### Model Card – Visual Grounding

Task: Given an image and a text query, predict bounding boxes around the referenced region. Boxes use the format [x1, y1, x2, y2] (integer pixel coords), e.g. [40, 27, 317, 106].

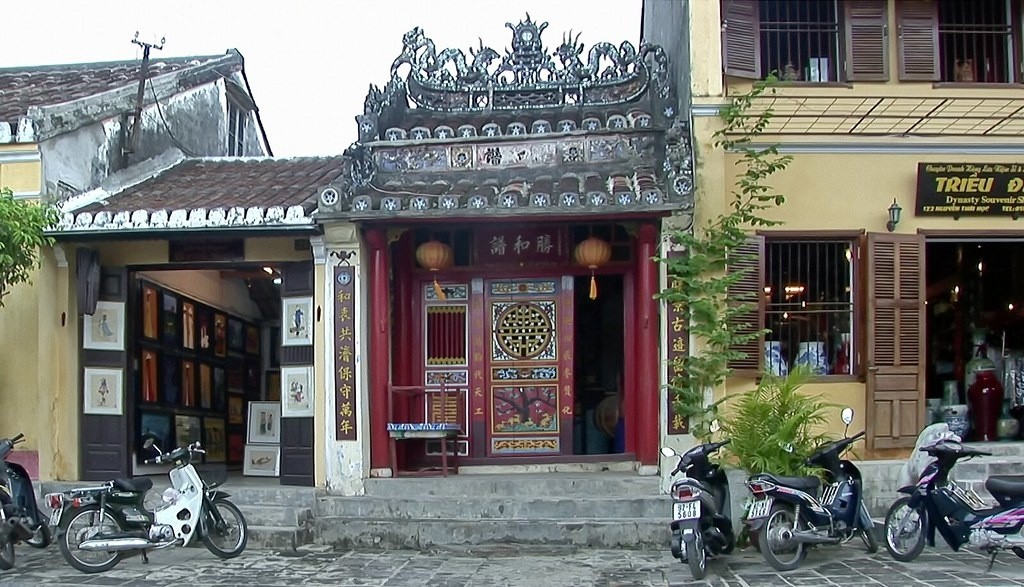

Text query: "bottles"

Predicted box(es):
[765, 333, 850, 376]
[925, 325, 1019, 443]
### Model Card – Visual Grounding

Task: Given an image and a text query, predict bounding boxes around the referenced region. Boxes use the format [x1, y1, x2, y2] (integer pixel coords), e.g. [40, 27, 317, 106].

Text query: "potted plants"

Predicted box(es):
[718, 367, 862, 555]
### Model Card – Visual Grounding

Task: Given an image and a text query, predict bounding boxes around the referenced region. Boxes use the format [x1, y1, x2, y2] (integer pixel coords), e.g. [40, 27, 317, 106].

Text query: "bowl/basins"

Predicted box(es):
[938, 405, 970, 418]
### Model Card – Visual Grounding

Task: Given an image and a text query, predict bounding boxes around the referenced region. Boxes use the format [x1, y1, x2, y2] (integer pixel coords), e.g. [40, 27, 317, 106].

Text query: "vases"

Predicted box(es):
[794, 341, 832, 374]
[836, 333, 850, 373]
[926, 329, 1024, 444]
[766, 340, 789, 377]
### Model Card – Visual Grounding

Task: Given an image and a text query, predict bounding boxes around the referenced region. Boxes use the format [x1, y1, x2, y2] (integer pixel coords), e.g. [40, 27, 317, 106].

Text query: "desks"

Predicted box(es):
[386, 380, 465, 478]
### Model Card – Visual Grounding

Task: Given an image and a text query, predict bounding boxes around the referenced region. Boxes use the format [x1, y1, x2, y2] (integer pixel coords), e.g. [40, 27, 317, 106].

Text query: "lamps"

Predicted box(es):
[885, 197, 902, 233]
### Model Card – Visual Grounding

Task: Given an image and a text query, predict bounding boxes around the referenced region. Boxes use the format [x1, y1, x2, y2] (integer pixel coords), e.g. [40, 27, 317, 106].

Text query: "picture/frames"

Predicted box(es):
[82, 278, 313, 477]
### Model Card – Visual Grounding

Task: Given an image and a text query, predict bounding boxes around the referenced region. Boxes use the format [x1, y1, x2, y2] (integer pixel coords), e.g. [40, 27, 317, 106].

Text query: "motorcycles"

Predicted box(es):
[883, 422, 1024, 573]
[0, 433, 53, 571]
[660, 417, 735, 582]
[740, 407, 879, 571]
[44, 436, 248, 574]
